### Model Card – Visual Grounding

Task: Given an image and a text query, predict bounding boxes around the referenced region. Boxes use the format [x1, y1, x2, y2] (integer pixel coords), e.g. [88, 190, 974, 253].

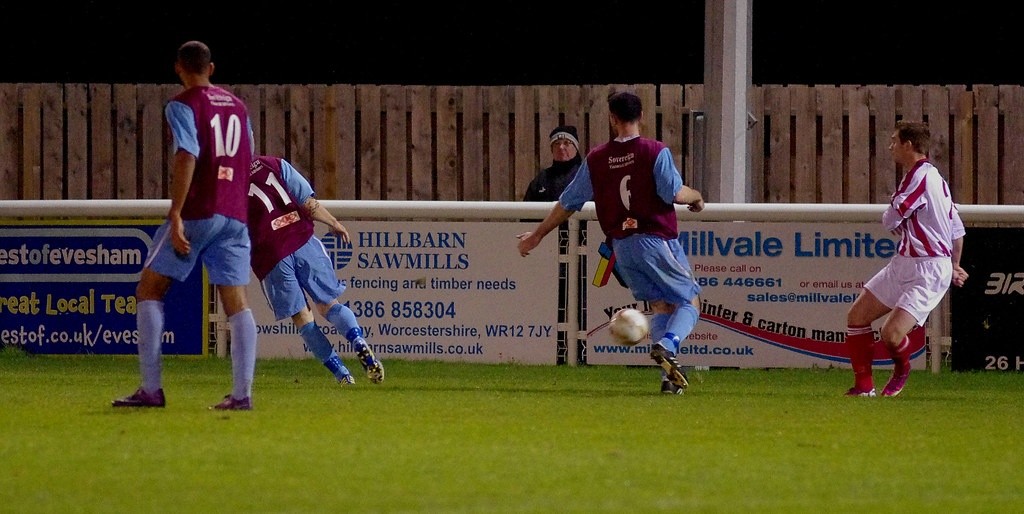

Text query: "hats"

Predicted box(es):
[549, 125, 578, 151]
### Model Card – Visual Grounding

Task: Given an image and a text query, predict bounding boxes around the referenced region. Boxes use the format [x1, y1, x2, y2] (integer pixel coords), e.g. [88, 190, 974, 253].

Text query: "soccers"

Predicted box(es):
[609, 307, 649, 348]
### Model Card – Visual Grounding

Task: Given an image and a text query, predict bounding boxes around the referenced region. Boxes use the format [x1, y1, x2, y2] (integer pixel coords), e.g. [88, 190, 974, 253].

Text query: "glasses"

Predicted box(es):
[551, 141, 573, 148]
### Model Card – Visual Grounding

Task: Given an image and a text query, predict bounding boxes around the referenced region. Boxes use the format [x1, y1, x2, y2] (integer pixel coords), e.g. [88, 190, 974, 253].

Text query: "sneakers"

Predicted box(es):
[648, 345, 689, 389]
[351, 338, 384, 383]
[843, 387, 876, 397]
[881, 364, 911, 397]
[209, 393, 252, 409]
[112, 387, 166, 409]
[338, 375, 356, 386]
[660, 379, 684, 394]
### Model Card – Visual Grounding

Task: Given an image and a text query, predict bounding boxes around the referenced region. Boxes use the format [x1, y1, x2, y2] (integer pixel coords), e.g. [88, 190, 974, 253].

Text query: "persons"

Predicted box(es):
[520, 124, 597, 365]
[248, 154, 385, 387]
[843, 119, 970, 398]
[111, 40, 258, 414]
[516, 92, 705, 395]
